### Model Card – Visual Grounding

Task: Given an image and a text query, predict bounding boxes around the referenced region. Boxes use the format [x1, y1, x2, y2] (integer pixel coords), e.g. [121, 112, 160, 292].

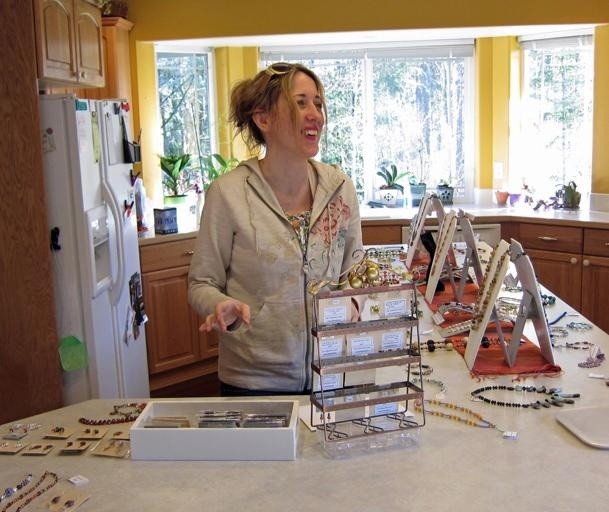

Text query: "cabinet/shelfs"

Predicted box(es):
[46, 14, 136, 140]
[32, 0, 108, 92]
[359, 217, 405, 247]
[508, 218, 609, 335]
[140, 232, 227, 393]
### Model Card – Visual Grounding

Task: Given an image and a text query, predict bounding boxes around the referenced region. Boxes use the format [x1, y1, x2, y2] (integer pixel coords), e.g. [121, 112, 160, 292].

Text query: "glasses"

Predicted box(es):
[258, 62, 290, 95]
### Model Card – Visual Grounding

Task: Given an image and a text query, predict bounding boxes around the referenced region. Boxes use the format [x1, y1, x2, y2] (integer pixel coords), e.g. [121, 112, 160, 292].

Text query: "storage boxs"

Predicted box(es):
[129, 400, 300, 462]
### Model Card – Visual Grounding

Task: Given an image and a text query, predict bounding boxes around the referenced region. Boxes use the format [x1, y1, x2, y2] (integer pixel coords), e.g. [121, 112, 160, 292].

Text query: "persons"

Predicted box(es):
[185, 61, 368, 396]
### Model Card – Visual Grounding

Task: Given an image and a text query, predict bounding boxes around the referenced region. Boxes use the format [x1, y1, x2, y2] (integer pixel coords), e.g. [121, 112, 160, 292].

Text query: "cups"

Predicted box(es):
[494, 190, 509, 210]
[508, 192, 522, 208]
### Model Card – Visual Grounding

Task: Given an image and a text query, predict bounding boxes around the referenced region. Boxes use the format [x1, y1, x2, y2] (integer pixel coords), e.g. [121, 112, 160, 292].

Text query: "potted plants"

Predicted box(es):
[157, 149, 193, 209]
[375, 162, 413, 209]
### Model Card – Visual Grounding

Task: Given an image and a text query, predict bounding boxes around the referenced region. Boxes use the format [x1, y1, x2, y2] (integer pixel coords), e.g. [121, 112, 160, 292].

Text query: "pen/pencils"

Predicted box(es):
[137, 128, 142, 144]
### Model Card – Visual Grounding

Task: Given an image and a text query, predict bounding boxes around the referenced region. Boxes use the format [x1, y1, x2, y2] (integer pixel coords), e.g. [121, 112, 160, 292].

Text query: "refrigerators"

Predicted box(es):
[38, 92, 153, 403]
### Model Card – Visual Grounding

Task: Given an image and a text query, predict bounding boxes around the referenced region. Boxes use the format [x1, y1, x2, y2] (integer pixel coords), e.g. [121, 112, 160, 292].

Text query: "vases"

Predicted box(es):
[436, 185, 456, 207]
[410, 182, 428, 208]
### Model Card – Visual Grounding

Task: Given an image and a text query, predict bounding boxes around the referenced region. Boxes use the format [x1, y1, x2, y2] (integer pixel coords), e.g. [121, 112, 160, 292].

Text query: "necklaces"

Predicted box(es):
[1, 470, 60, 511]
[364, 195, 605, 434]
[110, 401, 146, 415]
[0, 472, 33, 500]
[79, 416, 138, 427]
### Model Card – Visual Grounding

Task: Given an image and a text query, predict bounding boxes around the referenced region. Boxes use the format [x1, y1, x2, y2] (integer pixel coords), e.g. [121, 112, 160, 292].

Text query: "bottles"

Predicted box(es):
[194, 188, 205, 228]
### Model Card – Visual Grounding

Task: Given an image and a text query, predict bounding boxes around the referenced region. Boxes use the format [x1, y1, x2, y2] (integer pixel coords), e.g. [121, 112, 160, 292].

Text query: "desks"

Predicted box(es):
[0, 236, 608, 510]
